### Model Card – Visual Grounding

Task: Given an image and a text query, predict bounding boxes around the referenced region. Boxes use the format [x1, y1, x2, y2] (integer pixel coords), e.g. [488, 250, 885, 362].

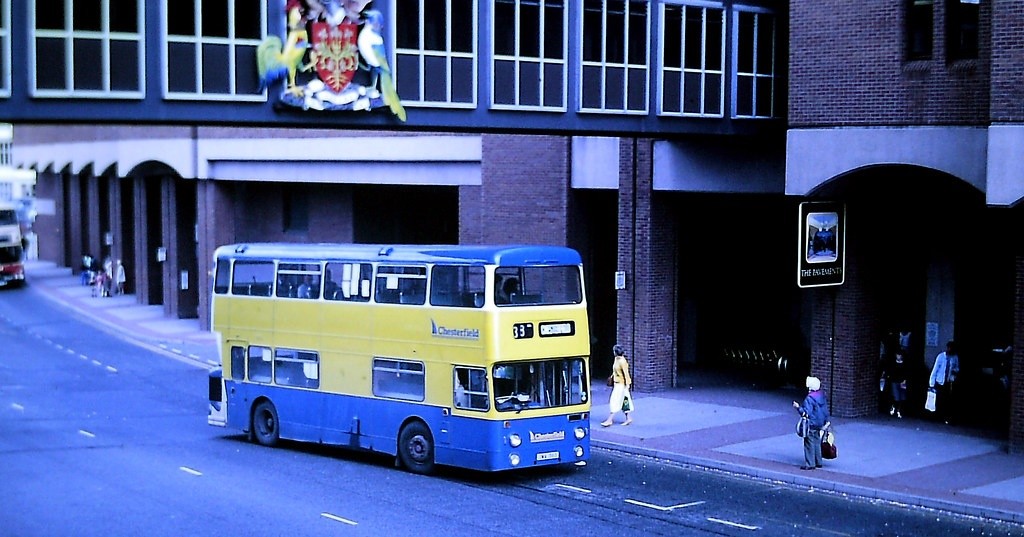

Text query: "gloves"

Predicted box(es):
[793, 401, 800, 408]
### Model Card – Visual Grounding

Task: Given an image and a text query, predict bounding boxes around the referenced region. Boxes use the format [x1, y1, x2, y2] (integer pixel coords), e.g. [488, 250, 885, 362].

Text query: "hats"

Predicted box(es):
[895, 348, 907, 357]
[805, 376, 821, 391]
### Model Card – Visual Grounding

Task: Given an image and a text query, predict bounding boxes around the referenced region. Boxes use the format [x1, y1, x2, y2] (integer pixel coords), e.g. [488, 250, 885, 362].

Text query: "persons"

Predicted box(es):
[793, 376, 831, 470]
[879, 323, 1013, 429]
[20, 235, 127, 299]
[455, 378, 473, 408]
[494, 275, 524, 307]
[298, 269, 344, 301]
[601, 345, 634, 427]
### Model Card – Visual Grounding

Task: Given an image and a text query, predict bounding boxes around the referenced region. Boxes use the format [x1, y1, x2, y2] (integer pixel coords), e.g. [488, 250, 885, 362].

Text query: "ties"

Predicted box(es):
[945, 356, 950, 382]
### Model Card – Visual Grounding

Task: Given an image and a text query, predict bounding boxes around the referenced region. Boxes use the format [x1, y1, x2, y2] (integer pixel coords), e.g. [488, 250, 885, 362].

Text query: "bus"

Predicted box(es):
[0, 208, 27, 288]
[205, 243, 593, 475]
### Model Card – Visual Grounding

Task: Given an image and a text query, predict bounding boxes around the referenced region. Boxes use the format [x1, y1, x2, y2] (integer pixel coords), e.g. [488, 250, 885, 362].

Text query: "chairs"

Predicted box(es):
[438, 291, 484, 307]
[249, 285, 255, 295]
[268, 284, 272, 296]
[333, 290, 345, 300]
[289, 282, 297, 297]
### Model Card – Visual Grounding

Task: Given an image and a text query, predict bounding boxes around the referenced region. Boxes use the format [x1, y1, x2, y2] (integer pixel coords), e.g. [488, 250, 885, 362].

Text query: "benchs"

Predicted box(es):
[400, 289, 426, 304]
[511, 289, 543, 303]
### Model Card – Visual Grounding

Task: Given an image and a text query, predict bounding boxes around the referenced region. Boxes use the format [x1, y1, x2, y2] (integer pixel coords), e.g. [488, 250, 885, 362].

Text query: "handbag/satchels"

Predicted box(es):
[607, 374, 614, 386]
[925, 388, 936, 411]
[795, 412, 810, 437]
[880, 374, 886, 391]
[622, 396, 630, 411]
[821, 430, 837, 459]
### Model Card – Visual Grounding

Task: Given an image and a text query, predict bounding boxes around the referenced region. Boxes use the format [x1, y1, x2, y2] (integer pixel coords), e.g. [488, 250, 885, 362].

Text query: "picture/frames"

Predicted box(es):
[797, 201, 846, 288]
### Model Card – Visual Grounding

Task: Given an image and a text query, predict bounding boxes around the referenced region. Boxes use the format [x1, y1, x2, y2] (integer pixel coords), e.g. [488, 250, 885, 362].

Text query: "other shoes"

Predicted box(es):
[621, 419, 632, 425]
[800, 465, 816, 470]
[815, 463, 823, 468]
[889, 404, 895, 415]
[600, 421, 614, 426]
[896, 411, 902, 420]
